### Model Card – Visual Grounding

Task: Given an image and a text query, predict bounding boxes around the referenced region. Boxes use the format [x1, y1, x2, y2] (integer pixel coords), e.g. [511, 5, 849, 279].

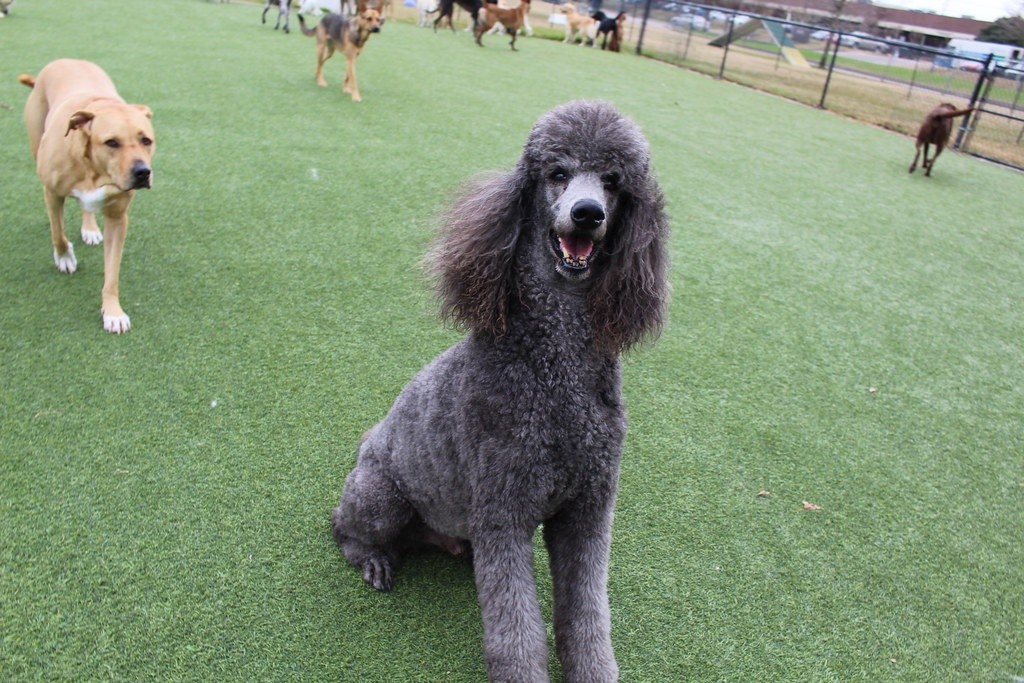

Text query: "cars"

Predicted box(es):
[670, 13, 710, 32]
[840, 32, 889, 54]
[664, 2, 844, 44]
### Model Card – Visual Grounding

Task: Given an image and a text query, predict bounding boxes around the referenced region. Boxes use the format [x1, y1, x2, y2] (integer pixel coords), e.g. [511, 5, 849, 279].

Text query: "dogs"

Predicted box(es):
[18, 59, 155, 335]
[331, 98, 671, 683]
[909, 101, 976, 178]
[262, 0, 627, 101]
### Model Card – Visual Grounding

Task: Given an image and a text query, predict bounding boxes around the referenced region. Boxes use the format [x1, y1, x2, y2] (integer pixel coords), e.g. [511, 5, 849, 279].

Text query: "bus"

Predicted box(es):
[944, 38, 1024, 78]
